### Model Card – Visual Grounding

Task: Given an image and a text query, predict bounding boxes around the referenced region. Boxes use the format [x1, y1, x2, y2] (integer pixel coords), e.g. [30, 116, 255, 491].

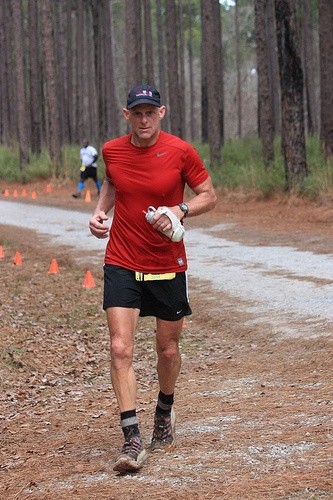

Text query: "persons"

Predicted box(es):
[72, 137, 102, 199]
[89, 84, 217, 473]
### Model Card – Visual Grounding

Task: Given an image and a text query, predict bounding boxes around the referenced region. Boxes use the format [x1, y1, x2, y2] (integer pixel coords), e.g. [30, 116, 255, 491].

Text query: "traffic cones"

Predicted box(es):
[84, 190, 91, 202]
[82, 270, 97, 288]
[4, 184, 52, 201]
[0, 245, 5, 257]
[47, 259, 62, 274]
[12, 251, 25, 266]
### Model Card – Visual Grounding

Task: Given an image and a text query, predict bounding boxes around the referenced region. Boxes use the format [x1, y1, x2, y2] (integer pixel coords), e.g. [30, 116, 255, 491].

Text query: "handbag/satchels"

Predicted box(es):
[134, 270, 177, 282]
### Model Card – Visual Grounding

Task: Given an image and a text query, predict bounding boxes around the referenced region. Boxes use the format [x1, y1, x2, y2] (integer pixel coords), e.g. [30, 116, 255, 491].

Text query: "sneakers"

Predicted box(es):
[151, 410, 177, 453]
[72, 192, 81, 199]
[113, 437, 149, 473]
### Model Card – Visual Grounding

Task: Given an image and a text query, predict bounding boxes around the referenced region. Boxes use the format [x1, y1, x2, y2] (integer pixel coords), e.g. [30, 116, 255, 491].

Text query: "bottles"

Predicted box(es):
[146, 206, 185, 242]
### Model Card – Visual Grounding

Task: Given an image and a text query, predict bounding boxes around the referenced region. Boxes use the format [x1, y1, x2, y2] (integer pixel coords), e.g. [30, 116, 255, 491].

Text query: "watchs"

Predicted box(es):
[177, 202, 189, 219]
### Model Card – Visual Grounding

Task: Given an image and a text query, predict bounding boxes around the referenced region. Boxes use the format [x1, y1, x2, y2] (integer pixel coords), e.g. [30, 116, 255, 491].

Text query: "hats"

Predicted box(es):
[126, 85, 162, 110]
